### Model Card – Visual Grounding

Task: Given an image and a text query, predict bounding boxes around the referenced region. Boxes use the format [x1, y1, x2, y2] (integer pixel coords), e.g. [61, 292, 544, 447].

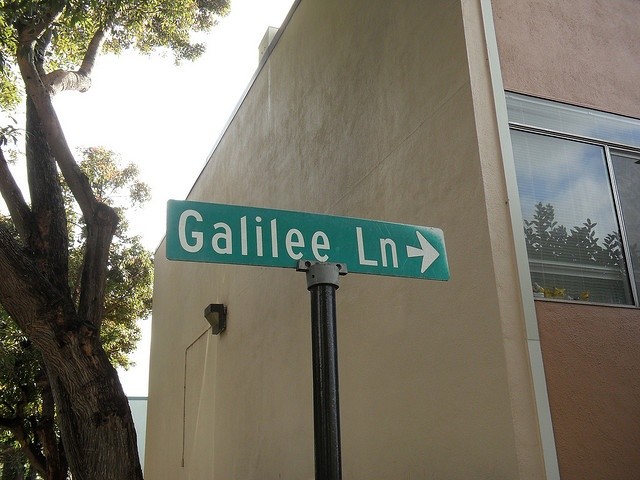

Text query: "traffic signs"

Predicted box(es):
[166, 199, 450, 282]
